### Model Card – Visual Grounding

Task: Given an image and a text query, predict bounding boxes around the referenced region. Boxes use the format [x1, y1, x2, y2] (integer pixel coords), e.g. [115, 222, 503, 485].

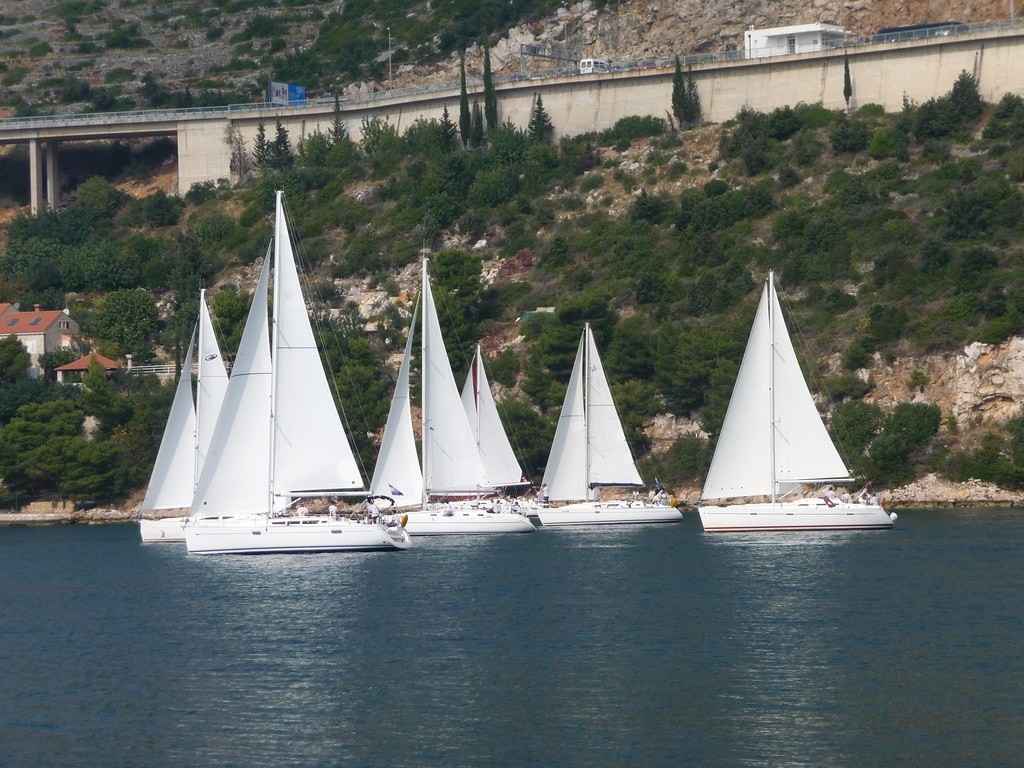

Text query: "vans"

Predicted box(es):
[578, 58, 607, 75]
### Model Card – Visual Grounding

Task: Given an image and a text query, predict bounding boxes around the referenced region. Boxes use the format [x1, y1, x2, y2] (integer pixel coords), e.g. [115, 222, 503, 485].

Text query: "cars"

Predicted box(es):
[608, 53, 718, 73]
[508, 69, 581, 83]
[842, 36, 872, 48]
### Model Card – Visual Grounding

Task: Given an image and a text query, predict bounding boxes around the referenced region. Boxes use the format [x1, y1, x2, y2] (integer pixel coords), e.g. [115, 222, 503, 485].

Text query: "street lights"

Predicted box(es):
[386, 27, 392, 90]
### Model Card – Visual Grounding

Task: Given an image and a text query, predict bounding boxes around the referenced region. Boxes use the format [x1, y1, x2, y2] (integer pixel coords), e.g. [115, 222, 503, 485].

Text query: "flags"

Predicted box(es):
[389, 484, 404, 495]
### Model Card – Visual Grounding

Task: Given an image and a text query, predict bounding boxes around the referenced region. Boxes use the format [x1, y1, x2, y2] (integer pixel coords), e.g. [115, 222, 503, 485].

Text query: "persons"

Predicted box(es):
[329, 502, 339, 521]
[283, 508, 289, 517]
[443, 484, 549, 517]
[296, 503, 308, 524]
[826, 487, 896, 508]
[363, 499, 379, 525]
[631, 477, 678, 509]
[385, 510, 399, 527]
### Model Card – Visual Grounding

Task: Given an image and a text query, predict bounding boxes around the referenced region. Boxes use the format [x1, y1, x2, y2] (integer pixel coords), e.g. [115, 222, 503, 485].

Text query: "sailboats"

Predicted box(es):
[698, 269, 898, 532]
[181, 190, 413, 554]
[363, 257, 538, 536]
[534, 321, 683, 528]
[134, 288, 267, 544]
